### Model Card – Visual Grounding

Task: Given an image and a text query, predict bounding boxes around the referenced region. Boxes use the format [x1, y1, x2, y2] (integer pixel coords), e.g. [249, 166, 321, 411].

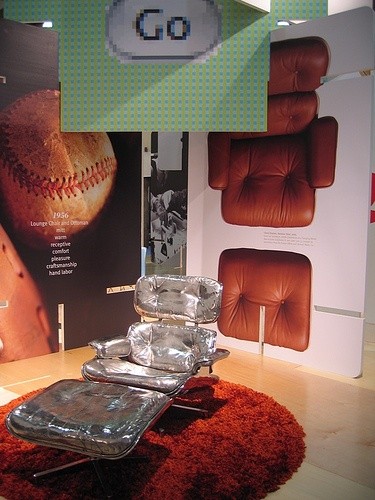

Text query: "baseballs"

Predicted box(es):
[0, 89, 118, 250]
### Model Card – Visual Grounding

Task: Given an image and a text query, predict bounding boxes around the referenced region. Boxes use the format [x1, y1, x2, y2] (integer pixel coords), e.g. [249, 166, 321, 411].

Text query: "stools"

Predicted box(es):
[212, 247, 314, 353]
[4, 378, 174, 490]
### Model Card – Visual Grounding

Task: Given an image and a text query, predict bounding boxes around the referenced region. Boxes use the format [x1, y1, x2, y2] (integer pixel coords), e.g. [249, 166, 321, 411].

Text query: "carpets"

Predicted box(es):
[1, 372, 307, 500]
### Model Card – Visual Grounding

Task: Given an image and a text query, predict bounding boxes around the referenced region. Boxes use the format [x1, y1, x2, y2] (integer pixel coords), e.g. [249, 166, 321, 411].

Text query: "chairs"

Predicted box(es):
[207, 37, 339, 230]
[80, 272, 222, 398]
